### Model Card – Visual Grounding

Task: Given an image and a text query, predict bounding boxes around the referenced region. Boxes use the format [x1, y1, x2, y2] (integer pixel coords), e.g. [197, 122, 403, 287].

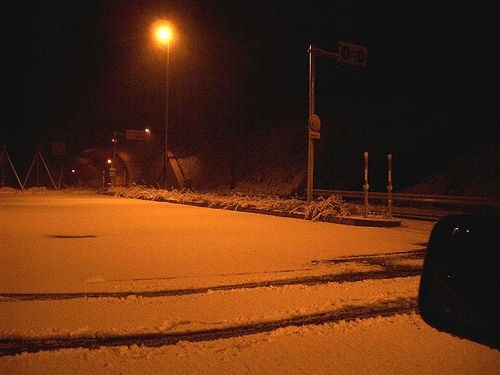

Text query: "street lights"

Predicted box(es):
[156, 21, 167, 189]
